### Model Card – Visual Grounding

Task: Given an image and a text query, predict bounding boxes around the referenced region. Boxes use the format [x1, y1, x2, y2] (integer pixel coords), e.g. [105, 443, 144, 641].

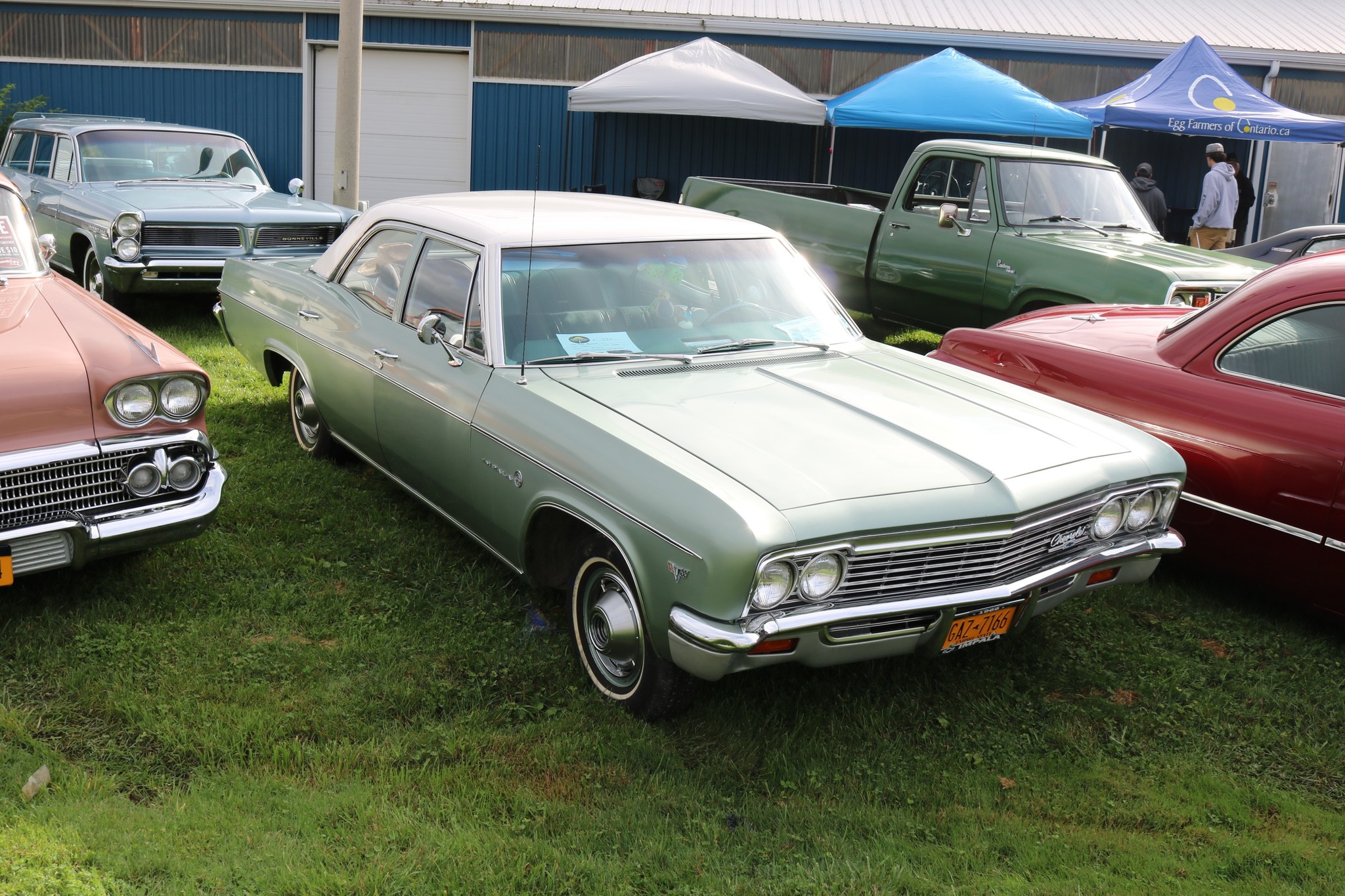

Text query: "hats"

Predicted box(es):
[1206, 143, 1224, 154]
[1136, 162, 1152, 175]
[1226, 153, 1239, 163]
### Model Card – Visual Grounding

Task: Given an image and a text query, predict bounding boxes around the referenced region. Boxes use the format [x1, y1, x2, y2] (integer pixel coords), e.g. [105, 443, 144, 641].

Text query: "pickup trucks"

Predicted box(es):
[677, 140, 1281, 333]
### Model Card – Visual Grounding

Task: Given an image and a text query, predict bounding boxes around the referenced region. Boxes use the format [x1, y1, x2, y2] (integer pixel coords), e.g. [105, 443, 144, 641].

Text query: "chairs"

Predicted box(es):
[446, 262, 715, 353]
[44, 150, 226, 184]
[634, 173, 671, 202]
[581, 183, 609, 197]
[920, 189, 1107, 230]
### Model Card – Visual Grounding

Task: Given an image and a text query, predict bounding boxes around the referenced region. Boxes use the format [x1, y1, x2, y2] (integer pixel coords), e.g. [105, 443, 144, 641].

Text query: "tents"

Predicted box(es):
[820, 45, 1096, 193]
[563, 36, 827, 193]
[1043, 35, 1344, 225]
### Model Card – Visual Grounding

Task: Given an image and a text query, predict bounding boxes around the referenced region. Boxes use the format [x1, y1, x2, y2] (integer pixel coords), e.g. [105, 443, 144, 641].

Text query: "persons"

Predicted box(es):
[1130, 143, 1255, 250]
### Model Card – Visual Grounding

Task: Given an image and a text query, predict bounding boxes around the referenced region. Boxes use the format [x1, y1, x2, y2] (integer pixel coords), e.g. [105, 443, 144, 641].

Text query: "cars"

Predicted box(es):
[921, 224, 1345, 582]
[214, 191, 1188, 707]
[1, 173, 229, 588]
[0, 111, 364, 311]
